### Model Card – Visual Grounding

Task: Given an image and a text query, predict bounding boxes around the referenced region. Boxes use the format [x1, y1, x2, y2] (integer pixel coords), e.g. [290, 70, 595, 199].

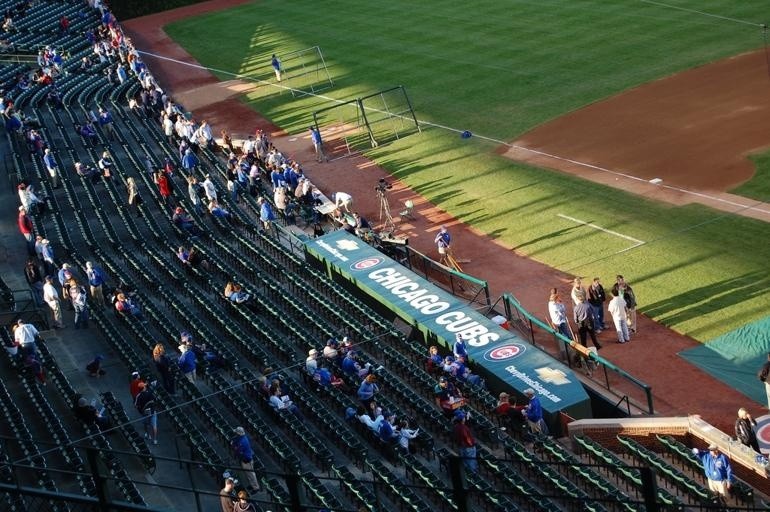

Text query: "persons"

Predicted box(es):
[759, 352, 770, 412]
[692, 445, 734, 505]
[233, 492, 256, 511]
[220, 479, 233, 511]
[735, 408, 761, 452]
[231, 427, 263, 494]
[549, 269, 638, 347]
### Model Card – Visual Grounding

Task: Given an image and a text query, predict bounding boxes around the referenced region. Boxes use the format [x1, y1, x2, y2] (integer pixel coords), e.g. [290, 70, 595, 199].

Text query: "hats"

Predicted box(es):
[442, 333, 464, 363]
[707, 443, 719, 451]
[523, 388, 535, 395]
[439, 376, 447, 383]
[499, 392, 510, 398]
[308, 348, 318, 356]
[372, 407, 395, 419]
[326, 338, 336, 347]
[453, 408, 467, 422]
[232, 426, 246, 436]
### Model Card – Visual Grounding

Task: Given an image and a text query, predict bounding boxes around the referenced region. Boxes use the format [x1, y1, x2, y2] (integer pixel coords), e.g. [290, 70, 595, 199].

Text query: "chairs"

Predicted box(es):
[0, 0, 770, 512]
